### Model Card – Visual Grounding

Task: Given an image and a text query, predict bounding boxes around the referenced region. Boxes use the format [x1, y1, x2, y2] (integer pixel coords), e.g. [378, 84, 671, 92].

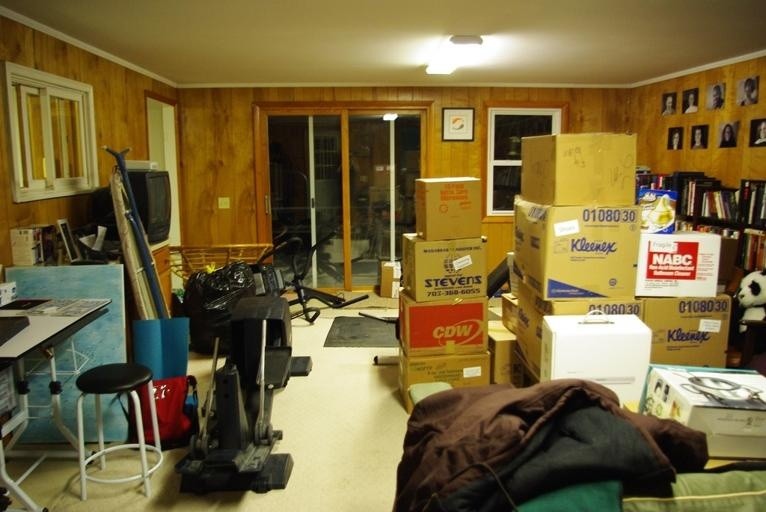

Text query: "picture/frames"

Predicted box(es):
[668, 126, 684, 150]
[692, 124, 710, 150]
[442, 107, 475, 141]
[720, 123, 739, 148]
[56, 218, 82, 264]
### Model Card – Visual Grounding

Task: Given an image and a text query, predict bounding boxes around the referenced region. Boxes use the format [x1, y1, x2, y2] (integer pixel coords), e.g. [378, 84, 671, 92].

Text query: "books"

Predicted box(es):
[632, 164, 766, 282]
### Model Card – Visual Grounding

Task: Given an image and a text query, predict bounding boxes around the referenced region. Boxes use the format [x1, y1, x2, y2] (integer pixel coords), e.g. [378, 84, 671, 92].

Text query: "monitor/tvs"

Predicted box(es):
[90, 171, 172, 244]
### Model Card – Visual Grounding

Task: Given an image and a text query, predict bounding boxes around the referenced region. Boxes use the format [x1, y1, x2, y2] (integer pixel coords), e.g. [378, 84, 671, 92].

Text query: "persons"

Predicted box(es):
[664, 78, 766, 149]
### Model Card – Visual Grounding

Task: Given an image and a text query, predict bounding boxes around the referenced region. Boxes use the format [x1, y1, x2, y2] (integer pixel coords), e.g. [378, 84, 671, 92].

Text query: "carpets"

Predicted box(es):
[323, 316, 402, 348]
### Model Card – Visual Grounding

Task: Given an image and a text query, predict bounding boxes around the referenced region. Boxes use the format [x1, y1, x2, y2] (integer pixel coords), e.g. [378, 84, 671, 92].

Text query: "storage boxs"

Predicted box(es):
[640, 233, 731, 368]
[487, 132, 640, 384]
[396, 177, 491, 415]
[379, 260, 403, 300]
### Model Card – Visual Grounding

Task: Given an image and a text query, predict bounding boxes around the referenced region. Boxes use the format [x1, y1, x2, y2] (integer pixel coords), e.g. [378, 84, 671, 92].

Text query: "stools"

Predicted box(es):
[75, 362, 165, 502]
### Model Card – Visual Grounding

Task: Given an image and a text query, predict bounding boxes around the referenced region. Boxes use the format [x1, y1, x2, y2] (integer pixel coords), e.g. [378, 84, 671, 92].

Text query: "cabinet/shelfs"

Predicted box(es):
[636, 173, 766, 359]
[4, 242, 172, 446]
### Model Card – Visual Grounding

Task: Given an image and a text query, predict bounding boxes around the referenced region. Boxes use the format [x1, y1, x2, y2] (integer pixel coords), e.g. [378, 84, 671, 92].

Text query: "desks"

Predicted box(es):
[0, 296, 113, 512]
